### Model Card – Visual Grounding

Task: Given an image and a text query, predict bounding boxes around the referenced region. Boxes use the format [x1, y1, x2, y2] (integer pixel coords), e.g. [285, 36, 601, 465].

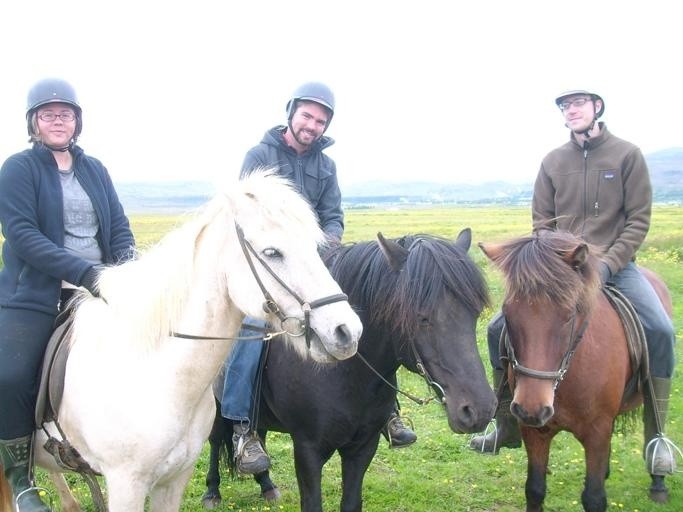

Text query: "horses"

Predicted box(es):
[200, 227, 500, 512]
[0, 163, 365, 512]
[477, 214, 674, 512]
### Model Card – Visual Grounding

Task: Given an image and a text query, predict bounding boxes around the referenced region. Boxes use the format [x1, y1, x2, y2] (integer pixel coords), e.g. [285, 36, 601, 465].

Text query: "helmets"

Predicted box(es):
[286, 82, 335, 132]
[25, 79, 82, 122]
[556, 90, 604, 117]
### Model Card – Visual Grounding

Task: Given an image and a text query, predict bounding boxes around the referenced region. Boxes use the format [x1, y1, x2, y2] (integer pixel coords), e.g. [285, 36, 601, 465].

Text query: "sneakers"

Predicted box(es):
[232, 417, 270, 476]
[381, 409, 417, 445]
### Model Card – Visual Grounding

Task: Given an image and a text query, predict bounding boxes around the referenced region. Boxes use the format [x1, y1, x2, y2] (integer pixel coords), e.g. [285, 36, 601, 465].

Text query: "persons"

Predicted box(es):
[471, 75, 677, 475]
[221, 83, 418, 474]
[0, 80, 135, 512]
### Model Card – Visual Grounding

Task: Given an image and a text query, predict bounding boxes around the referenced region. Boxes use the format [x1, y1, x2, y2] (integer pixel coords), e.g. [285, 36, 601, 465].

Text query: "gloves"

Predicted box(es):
[80, 266, 100, 297]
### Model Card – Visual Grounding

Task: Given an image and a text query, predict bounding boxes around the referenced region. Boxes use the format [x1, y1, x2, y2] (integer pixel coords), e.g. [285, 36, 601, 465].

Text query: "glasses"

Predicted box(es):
[38, 112, 75, 122]
[559, 97, 591, 109]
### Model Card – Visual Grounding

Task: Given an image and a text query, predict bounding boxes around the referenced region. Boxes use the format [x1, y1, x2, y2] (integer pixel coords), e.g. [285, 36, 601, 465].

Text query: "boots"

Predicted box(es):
[469, 368, 521, 451]
[642, 376, 675, 476]
[1, 435, 50, 511]
